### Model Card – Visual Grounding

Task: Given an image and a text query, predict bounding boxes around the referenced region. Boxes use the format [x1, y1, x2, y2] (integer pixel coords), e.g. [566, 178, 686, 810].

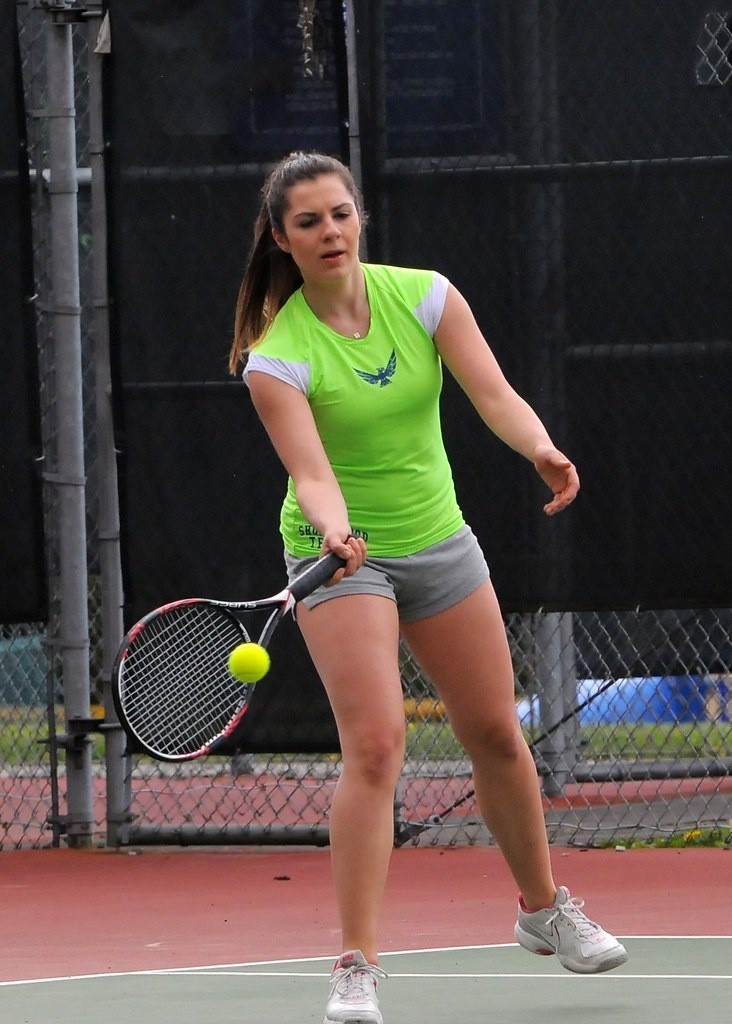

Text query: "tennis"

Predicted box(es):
[225, 644, 273, 682]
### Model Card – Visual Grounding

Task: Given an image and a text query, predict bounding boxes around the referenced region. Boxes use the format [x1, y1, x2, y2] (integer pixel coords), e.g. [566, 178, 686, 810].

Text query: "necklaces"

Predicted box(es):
[305, 290, 368, 339]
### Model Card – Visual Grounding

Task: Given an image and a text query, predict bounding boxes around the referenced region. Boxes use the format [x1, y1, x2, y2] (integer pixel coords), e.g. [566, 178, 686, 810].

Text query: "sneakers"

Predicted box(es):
[513, 886, 628, 974]
[324, 950, 389, 1024]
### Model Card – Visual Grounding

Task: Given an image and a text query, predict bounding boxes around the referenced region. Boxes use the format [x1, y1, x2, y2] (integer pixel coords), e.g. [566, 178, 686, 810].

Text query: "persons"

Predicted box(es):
[227, 151, 632, 1024]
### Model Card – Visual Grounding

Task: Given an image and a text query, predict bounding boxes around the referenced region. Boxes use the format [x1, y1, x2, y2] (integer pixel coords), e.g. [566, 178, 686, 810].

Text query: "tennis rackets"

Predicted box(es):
[110, 540, 373, 764]
